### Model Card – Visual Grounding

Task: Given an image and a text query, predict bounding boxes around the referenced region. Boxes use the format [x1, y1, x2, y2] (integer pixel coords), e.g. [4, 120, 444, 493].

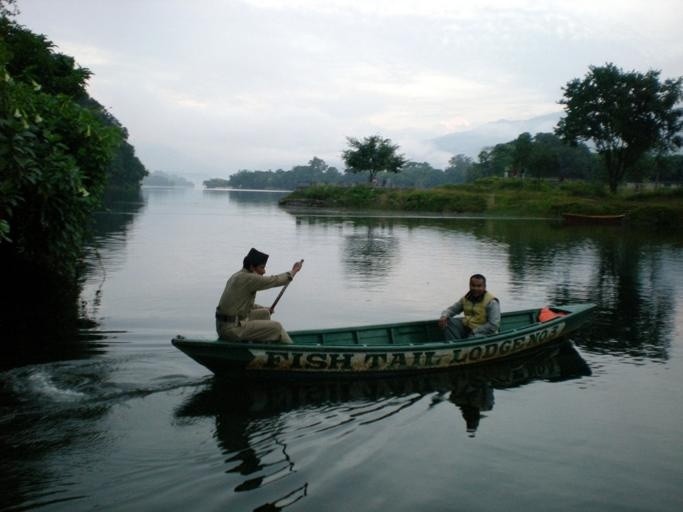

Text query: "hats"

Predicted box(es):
[247, 248, 270, 265]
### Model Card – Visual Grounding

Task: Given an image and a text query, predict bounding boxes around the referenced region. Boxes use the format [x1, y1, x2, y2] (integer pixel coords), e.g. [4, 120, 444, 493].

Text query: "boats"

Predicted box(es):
[171, 304, 596, 381]
[175, 344, 591, 414]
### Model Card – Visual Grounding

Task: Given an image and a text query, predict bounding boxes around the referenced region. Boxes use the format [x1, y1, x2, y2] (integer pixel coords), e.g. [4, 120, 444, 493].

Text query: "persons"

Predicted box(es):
[438, 274, 500, 340]
[215, 249, 303, 344]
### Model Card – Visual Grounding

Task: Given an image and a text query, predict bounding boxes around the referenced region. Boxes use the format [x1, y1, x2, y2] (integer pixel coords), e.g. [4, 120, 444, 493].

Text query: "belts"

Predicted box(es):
[215, 312, 248, 323]
